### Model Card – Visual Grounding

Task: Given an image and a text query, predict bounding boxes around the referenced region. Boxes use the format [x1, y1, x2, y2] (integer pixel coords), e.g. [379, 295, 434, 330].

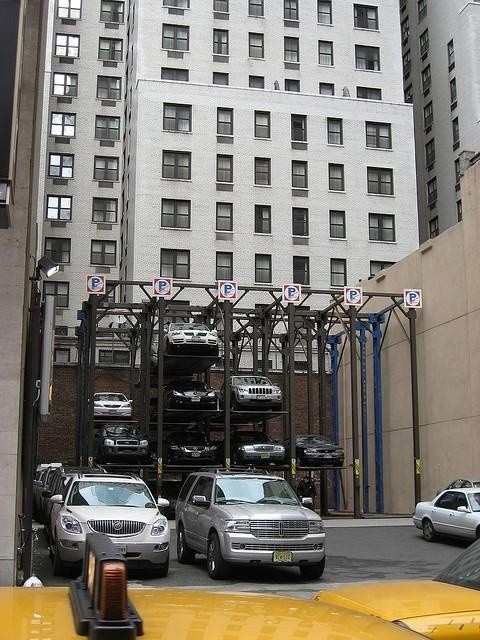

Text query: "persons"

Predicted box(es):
[297, 475, 317, 510]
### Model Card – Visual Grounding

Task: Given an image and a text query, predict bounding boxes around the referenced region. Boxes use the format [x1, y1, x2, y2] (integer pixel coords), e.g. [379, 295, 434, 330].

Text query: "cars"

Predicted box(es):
[220, 375, 283, 411]
[296, 434, 345, 466]
[163, 380, 217, 410]
[226, 431, 286, 464]
[164, 323, 219, 356]
[175, 469, 326, 578]
[88, 392, 134, 420]
[166, 431, 216, 465]
[94, 424, 149, 461]
[32, 463, 170, 577]
[412, 479, 480, 542]
